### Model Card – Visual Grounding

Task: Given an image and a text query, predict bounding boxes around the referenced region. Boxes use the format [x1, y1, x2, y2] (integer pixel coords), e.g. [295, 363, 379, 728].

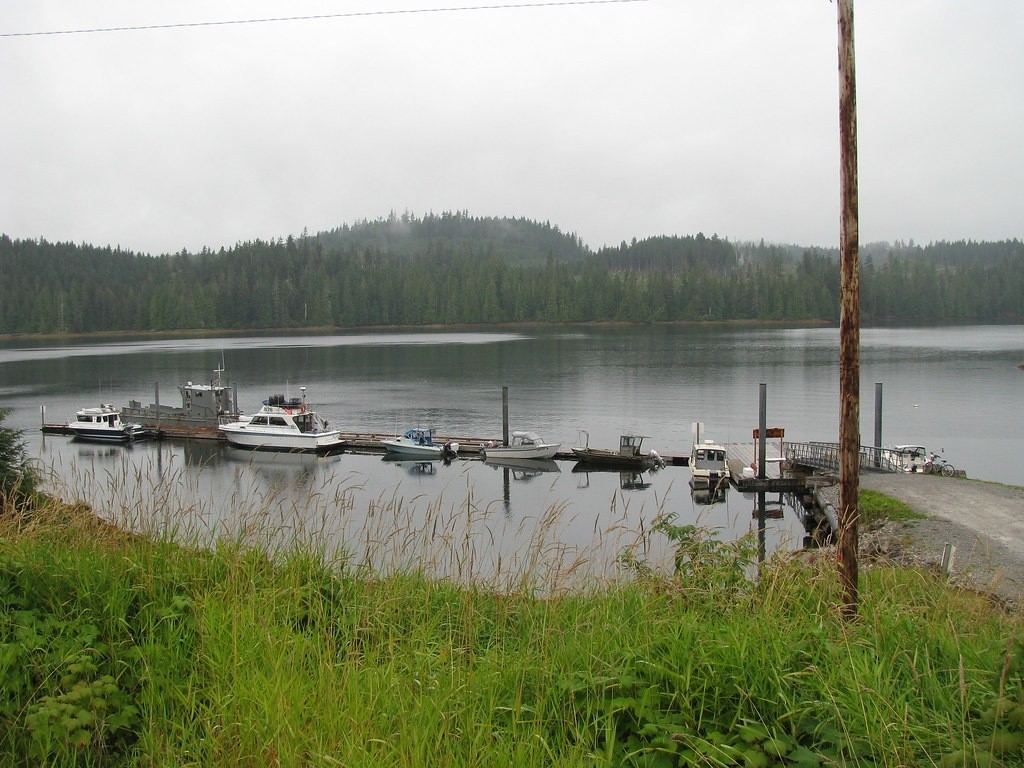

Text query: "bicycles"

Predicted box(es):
[923, 452, 955, 478]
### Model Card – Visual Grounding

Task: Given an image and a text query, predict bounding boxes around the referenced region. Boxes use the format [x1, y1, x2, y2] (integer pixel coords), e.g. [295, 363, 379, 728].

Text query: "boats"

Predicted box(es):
[121, 347, 245, 426]
[380, 452, 452, 477]
[884, 443, 930, 472]
[380, 428, 460, 456]
[484, 431, 561, 458]
[571, 462, 653, 491]
[218, 380, 346, 452]
[688, 440, 730, 478]
[69, 377, 143, 442]
[571, 430, 663, 465]
[483, 457, 561, 480]
[687, 475, 730, 505]
